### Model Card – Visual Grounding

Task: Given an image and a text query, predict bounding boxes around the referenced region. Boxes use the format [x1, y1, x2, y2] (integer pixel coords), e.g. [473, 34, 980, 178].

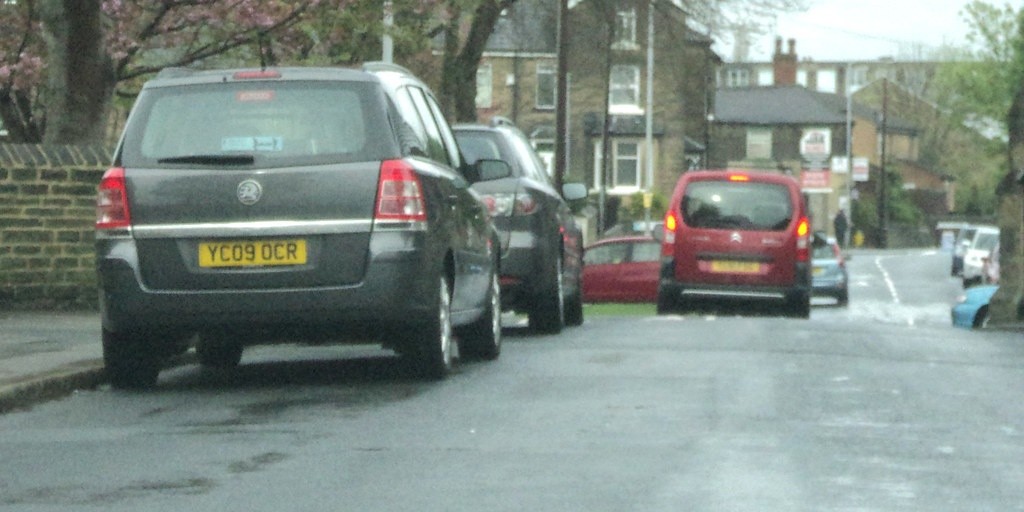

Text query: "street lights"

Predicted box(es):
[843, 74, 890, 246]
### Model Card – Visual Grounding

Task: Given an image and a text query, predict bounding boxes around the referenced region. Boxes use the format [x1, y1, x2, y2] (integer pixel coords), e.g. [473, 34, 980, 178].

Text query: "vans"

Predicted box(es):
[657, 170, 828, 318]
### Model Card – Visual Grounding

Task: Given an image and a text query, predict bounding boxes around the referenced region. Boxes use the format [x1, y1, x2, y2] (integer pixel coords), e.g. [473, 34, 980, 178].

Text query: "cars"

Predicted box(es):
[951, 226, 977, 275]
[961, 227, 999, 289]
[981, 242, 999, 286]
[951, 285, 999, 329]
[581, 238, 662, 304]
[811, 237, 850, 306]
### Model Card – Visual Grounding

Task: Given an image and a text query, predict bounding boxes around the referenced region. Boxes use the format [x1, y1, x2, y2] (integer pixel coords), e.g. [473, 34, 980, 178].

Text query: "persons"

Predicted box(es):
[604, 196, 621, 230]
[833, 210, 847, 246]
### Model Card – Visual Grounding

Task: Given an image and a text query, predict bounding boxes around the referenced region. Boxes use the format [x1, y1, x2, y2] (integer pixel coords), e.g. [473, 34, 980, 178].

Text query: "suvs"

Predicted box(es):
[96, 60, 513, 390]
[451, 115, 585, 334]
